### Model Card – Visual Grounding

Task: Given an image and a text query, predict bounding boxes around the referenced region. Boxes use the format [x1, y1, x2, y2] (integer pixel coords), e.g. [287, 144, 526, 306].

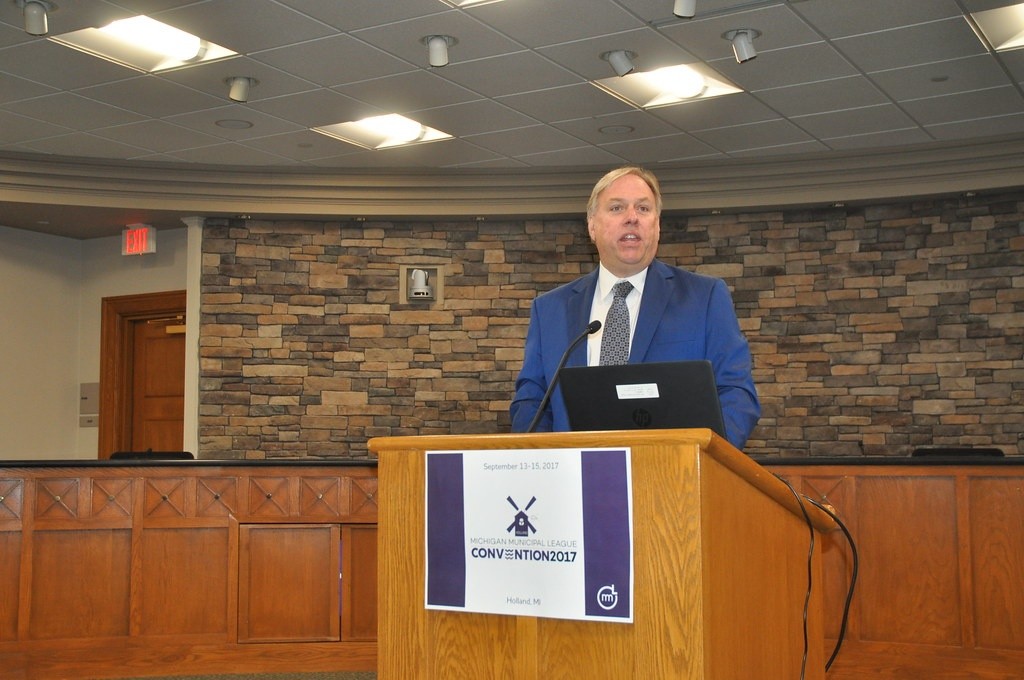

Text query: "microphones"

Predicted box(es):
[526, 321, 602, 433]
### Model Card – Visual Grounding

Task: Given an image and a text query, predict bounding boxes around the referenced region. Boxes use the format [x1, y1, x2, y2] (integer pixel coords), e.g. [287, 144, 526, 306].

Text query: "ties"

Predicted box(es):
[599, 281, 634, 366]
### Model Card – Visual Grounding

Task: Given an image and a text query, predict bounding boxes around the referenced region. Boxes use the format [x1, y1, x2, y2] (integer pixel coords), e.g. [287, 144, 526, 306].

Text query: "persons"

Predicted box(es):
[508, 166, 762, 453]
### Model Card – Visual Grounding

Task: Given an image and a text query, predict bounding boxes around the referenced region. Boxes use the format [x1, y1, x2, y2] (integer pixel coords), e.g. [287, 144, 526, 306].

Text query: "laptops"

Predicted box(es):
[559, 361, 727, 441]
